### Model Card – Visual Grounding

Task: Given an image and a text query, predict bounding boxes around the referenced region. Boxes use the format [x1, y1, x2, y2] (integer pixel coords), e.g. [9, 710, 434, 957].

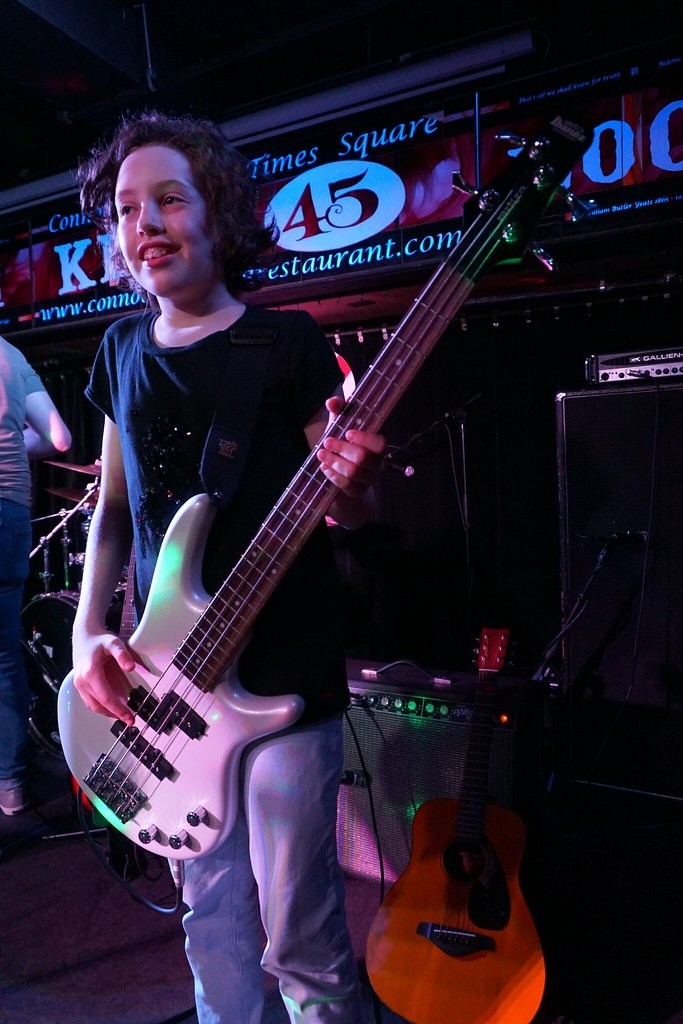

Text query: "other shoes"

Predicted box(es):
[0, 787, 29, 817]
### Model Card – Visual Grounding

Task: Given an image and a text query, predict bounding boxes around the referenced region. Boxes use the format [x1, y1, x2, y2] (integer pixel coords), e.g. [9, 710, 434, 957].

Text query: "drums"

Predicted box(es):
[21, 588, 81, 762]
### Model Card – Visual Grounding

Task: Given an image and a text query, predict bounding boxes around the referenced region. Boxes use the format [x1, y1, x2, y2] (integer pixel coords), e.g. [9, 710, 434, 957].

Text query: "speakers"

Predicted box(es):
[335, 658, 554, 906]
[550, 383, 683, 804]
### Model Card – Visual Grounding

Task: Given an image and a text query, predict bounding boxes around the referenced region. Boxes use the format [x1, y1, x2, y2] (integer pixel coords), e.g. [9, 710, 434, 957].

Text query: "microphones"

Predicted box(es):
[340, 769, 372, 788]
[611, 528, 650, 544]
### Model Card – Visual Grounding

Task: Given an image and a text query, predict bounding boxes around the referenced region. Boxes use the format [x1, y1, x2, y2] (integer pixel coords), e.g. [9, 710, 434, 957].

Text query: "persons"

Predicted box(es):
[75, 117, 389, 1024]
[0, 331, 74, 816]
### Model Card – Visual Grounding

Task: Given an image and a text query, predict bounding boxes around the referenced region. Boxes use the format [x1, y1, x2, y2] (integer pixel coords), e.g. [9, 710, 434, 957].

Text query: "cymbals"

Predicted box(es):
[43, 481, 100, 506]
[42, 458, 102, 478]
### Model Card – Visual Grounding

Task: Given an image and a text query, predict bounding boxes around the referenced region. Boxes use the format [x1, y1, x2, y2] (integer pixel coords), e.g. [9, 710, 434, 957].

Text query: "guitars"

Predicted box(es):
[54, 104, 606, 871]
[361, 618, 553, 1024]
